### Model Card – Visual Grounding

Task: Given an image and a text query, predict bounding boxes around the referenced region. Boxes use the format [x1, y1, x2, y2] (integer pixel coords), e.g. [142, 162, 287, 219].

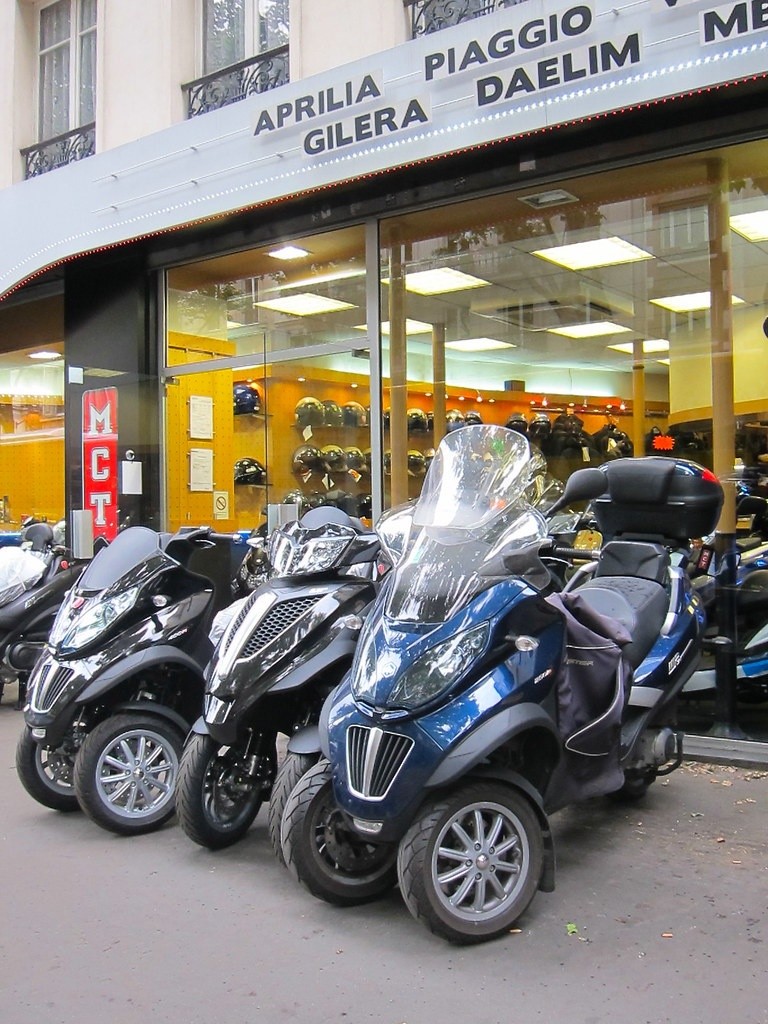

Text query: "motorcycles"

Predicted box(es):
[542, 495, 768, 710]
[281, 424, 725, 946]
[0, 516, 270, 835]
[176, 505, 395, 848]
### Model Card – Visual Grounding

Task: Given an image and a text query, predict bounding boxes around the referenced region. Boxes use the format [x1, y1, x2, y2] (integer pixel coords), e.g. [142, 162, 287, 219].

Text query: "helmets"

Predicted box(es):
[234, 382, 264, 414]
[283, 397, 552, 517]
[234, 458, 266, 485]
